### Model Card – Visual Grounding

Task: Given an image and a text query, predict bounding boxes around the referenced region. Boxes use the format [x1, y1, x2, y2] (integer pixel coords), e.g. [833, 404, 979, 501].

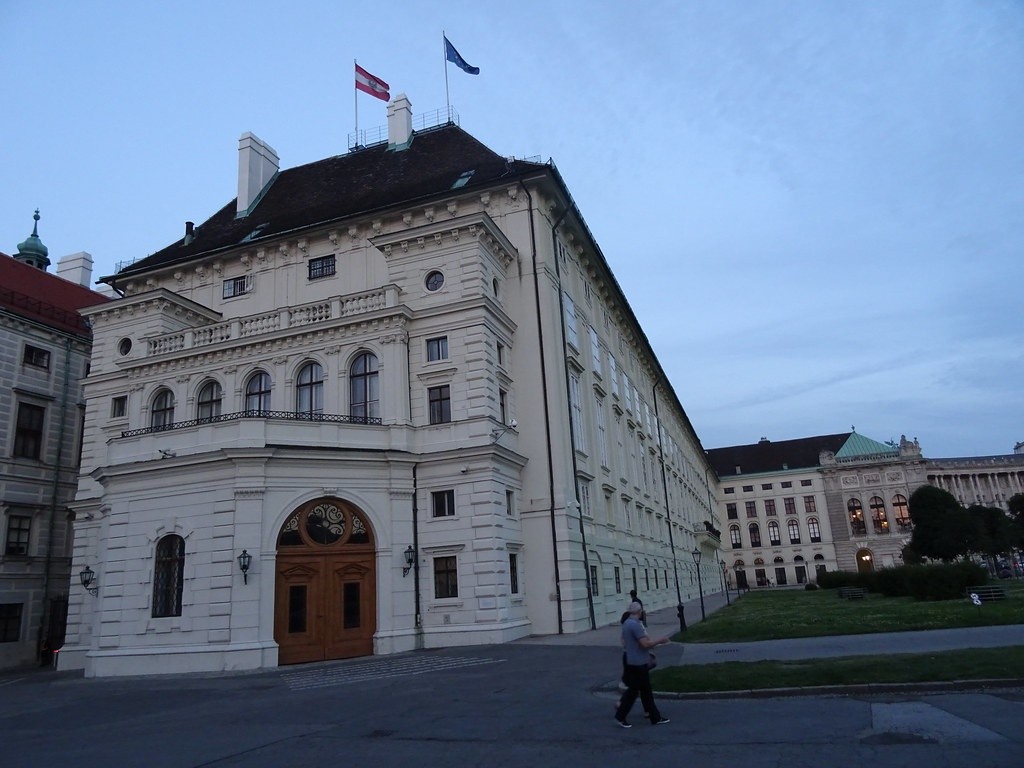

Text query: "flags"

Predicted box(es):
[355, 66, 390, 102]
[445, 36, 480, 76]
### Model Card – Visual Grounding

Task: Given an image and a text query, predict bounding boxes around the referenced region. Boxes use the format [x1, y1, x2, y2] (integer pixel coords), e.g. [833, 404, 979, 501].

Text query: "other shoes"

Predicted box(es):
[643, 713, 650, 719]
[619, 683, 629, 689]
[657, 717, 670, 724]
[613, 716, 632, 727]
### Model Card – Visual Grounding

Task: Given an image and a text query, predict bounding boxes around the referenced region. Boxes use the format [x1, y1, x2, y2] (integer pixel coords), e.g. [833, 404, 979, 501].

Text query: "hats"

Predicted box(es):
[627, 602, 641, 612]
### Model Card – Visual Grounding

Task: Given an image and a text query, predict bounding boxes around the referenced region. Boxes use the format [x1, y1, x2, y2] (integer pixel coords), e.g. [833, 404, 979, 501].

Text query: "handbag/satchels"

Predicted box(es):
[648, 650, 657, 669]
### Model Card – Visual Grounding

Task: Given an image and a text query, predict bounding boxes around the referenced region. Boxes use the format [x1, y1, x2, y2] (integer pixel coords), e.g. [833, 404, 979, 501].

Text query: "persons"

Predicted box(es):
[614, 590, 670, 729]
[767, 578, 771, 587]
[771, 576, 776, 588]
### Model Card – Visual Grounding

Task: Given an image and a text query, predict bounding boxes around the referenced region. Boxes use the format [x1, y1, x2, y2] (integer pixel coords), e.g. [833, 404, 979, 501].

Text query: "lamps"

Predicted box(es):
[403, 545, 415, 578]
[80, 565, 98, 598]
[237, 548, 252, 585]
